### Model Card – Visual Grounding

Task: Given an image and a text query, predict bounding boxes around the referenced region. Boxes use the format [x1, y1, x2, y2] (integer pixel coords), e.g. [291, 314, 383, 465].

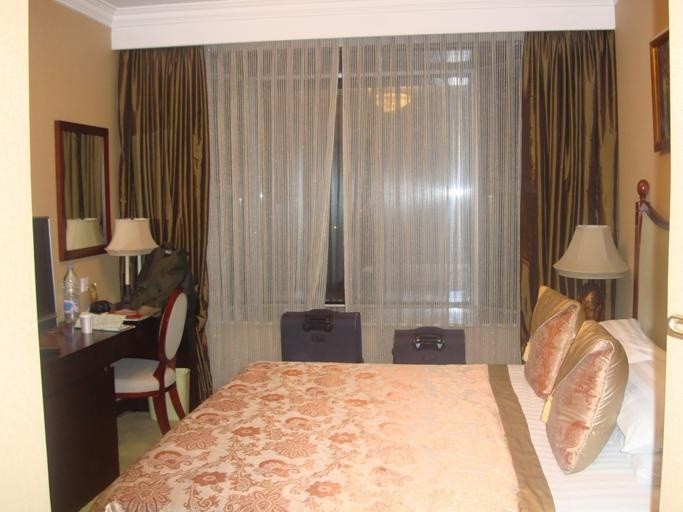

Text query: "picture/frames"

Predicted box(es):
[648, 29, 670, 153]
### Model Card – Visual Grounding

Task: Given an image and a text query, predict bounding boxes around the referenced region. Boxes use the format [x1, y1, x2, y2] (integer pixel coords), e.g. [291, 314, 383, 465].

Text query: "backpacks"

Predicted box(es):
[132, 243, 199, 312]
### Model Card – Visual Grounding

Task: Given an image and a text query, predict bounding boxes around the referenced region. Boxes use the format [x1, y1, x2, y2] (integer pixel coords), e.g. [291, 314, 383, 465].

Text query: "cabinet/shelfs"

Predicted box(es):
[39, 302, 202, 512]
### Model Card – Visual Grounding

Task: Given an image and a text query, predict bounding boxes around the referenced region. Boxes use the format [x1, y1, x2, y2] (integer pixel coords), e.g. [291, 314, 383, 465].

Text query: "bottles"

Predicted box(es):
[63, 263, 80, 324]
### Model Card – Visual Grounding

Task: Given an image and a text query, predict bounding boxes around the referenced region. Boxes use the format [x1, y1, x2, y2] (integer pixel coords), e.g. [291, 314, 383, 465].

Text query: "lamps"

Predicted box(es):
[553, 223, 629, 321]
[65, 212, 106, 250]
[105, 217, 160, 309]
[368, 86, 412, 113]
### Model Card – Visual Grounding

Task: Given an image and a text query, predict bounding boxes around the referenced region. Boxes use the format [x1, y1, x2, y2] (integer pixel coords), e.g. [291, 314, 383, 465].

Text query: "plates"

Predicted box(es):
[123, 315, 151, 322]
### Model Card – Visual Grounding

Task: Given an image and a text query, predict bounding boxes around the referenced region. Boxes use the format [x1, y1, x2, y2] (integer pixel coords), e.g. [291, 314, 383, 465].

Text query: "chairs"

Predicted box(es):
[110, 285, 189, 434]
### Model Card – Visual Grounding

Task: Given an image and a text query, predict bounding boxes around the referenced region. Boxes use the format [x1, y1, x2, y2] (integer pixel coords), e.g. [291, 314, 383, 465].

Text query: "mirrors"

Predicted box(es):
[54, 119, 112, 262]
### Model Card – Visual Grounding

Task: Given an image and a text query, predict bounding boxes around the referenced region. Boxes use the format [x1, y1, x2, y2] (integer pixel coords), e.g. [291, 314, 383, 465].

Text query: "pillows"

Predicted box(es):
[539, 318, 630, 477]
[597, 317, 667, 363]
[614, 362, 666, 454]
[522, 284, 579, 398]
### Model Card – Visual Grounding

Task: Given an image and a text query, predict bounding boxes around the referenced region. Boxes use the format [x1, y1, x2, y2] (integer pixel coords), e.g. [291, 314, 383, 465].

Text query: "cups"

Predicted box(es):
[79, 315, 93, 335]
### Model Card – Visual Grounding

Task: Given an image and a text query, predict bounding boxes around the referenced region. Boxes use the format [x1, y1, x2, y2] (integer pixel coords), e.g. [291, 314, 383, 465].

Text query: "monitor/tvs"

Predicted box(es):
[33, 216, 57, 323]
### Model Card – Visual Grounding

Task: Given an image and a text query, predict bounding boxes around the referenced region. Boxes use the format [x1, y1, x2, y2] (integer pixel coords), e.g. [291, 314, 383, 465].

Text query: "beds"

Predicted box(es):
[77, 179, 669, 512]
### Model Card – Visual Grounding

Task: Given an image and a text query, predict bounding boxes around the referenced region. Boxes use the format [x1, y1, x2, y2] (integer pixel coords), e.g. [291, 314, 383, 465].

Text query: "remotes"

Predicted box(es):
[40, 345, 61, 352]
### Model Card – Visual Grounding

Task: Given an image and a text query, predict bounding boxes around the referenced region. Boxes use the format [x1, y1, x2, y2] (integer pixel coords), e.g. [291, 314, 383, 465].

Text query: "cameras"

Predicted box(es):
[90, 301, 110, 313]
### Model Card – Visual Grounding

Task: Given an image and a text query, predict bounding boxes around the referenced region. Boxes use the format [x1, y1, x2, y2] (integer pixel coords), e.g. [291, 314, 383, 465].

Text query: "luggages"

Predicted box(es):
[280, 308, 363, 364]
[393, 326, 466, 365]
